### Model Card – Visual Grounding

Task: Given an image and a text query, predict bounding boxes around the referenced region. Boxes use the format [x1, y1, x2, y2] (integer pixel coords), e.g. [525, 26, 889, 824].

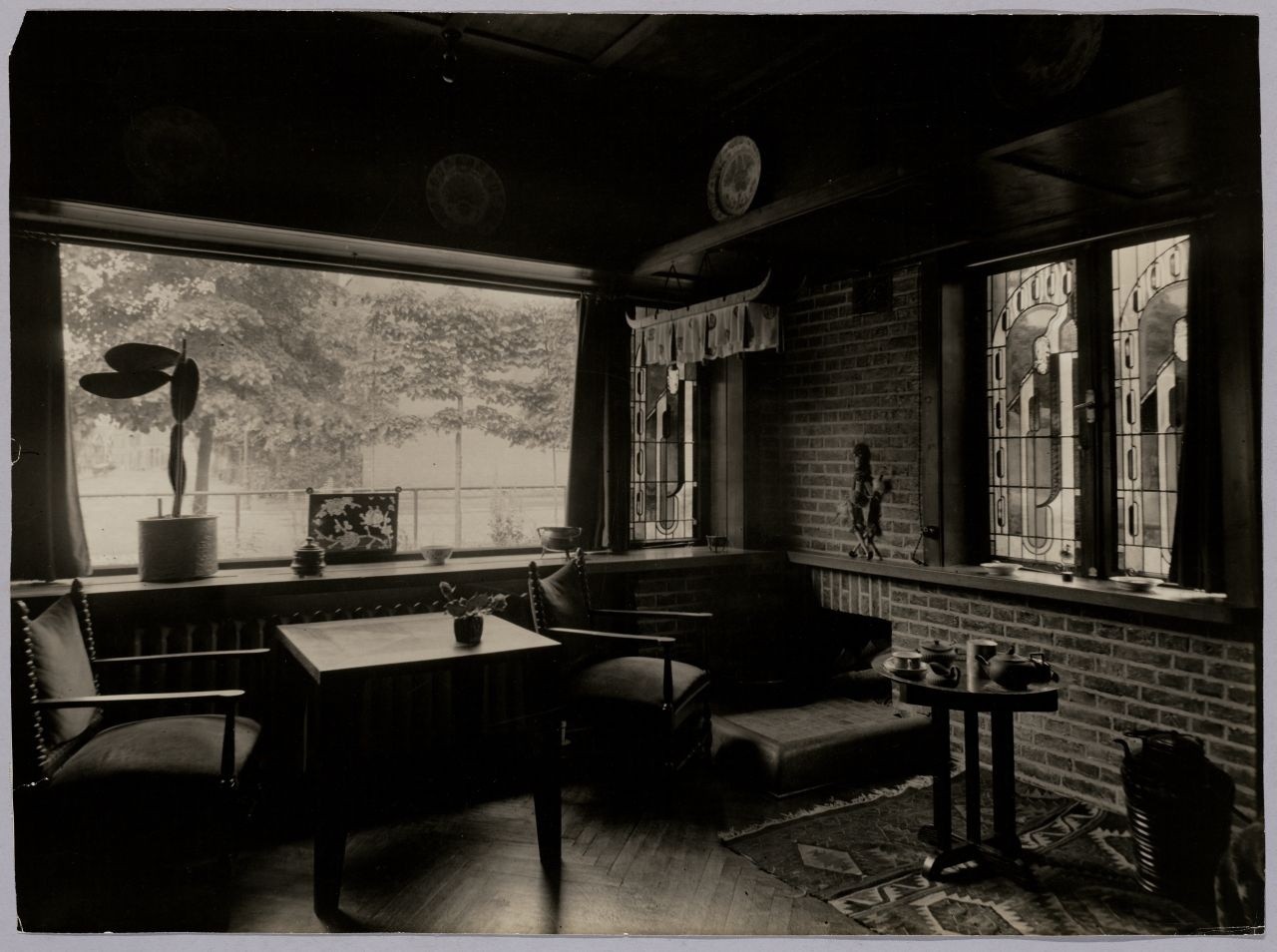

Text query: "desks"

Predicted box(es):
[278, 613, 565, 920]
[872, 650, 1061, 880]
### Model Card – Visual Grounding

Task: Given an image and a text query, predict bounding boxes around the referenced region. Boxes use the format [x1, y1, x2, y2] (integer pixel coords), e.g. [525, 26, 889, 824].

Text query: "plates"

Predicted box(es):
[882, 656, 929, 674]
[1110, 576, 1165, 592]
[981, 563, 1023, 575]
[706, 136, 761, 222]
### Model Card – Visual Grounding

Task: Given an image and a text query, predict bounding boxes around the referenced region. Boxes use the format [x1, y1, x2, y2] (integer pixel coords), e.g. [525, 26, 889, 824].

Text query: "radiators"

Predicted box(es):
[129, 592, 532, 772]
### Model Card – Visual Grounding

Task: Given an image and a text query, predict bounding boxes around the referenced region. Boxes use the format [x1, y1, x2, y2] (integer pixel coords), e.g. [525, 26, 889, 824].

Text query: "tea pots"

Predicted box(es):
[914, 639, 963, 669]
[1026, 652, 1051, 685]
[926, 663, 961, 688]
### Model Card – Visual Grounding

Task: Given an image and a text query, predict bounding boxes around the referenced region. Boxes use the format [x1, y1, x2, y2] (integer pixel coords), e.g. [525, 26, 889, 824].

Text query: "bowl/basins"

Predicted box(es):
[420, 546, 455, 565]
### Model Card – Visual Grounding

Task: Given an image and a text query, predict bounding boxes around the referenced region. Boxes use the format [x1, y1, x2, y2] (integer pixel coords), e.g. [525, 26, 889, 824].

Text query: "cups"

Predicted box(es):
[891, 651, 923, 670]
[975, 646, 1036, 690]
[967, 639, 998, 679]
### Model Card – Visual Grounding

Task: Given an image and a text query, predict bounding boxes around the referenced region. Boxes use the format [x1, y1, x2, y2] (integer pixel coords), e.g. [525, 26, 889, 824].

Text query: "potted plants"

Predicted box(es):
[79, 339, 222, 584]
[439, 578, 508, 648]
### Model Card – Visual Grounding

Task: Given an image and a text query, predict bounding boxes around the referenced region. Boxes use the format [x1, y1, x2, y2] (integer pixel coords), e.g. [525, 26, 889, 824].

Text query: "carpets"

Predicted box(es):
[718, 769, 1233, 936]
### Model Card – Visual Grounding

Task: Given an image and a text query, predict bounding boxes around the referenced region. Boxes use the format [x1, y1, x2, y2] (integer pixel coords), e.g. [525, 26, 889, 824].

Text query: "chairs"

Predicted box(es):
[17, 578, 271, 931]
[528, 548, 716, 782]
